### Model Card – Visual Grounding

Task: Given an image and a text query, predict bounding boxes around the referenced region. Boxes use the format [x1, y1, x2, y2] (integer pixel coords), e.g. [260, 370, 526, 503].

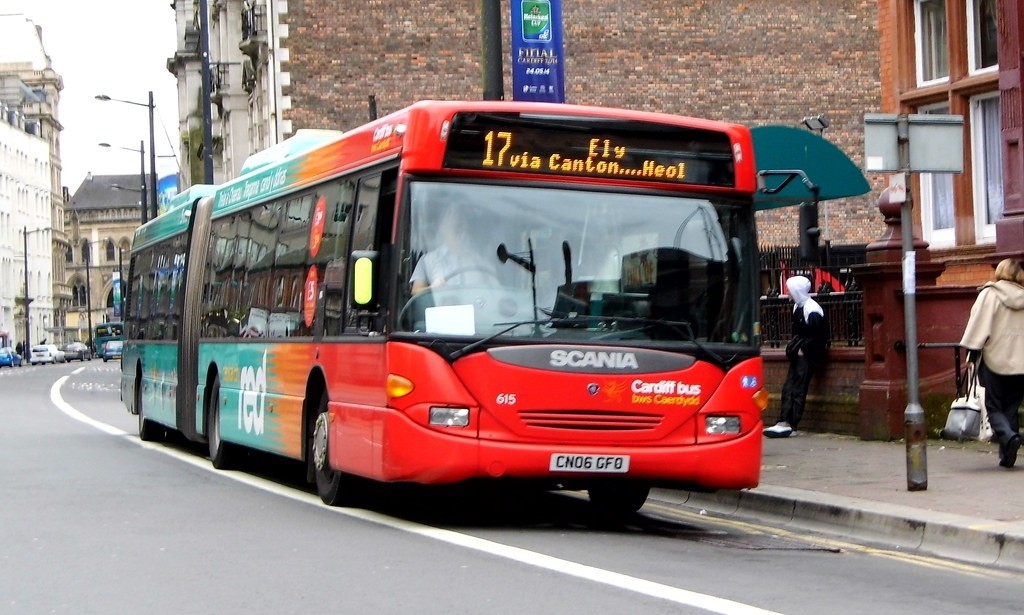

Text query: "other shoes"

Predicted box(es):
[762, 424, 793, 438]
[1000, 435, 1024, 467]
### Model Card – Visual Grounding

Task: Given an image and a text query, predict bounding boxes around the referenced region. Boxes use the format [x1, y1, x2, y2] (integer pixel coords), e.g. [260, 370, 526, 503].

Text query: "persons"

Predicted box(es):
[763, 276, 828, 438]
[409, 206, 501, 296]
[959, 258, 1024, 468]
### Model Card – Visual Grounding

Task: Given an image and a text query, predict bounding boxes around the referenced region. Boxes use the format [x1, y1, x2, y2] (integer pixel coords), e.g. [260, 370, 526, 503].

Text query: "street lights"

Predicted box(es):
[98, 140, 148, 226]
[22, 228, 68, 362]
[94, 91, 159, 219]
[86, 237, 111, 352]
[112, 180, 158, 226]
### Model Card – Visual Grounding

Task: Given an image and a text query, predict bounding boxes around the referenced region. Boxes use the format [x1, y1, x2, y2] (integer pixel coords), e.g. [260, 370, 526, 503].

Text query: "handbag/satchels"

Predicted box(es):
[942, 363, 980, 440]
[966, 364, 994, 443]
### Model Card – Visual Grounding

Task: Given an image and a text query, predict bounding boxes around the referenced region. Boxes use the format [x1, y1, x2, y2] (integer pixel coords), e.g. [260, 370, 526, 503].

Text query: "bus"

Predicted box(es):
[118, 98, 823, 509]
[92, 319, 123, 357]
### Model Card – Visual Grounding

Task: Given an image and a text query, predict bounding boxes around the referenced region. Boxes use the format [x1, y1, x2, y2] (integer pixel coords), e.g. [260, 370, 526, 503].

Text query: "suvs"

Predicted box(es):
[103, 340, 124, 366]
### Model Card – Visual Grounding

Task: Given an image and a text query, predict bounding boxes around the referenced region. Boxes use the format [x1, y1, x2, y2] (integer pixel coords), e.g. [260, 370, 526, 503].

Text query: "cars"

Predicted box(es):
[0, 347, 25, 367]
[63, 343, 93, 362]
[29, 344, 66, 364]
